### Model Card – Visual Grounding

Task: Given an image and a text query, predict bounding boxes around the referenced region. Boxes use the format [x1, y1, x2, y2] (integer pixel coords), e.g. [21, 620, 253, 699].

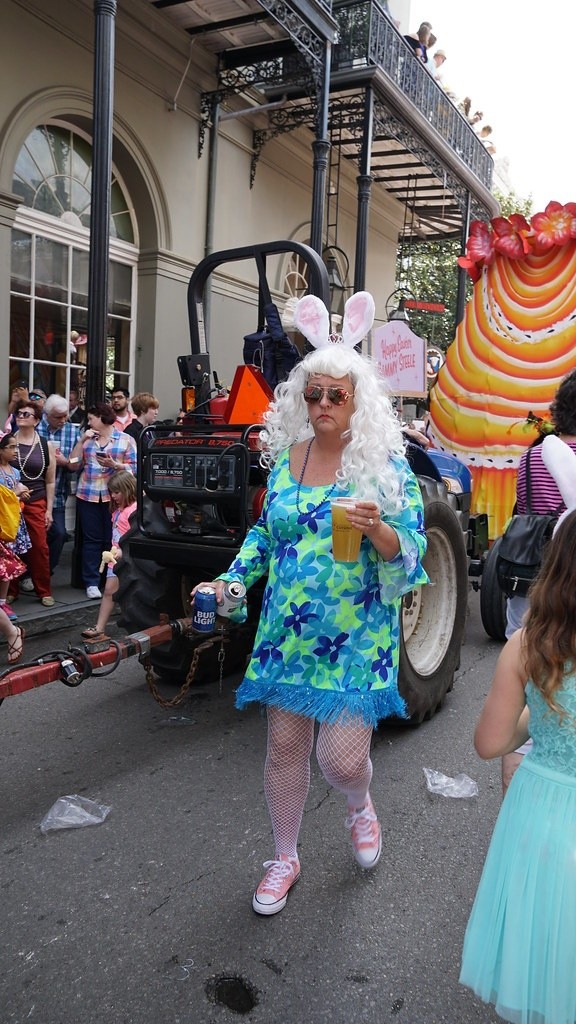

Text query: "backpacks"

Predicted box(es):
[497, 446, 565, 599]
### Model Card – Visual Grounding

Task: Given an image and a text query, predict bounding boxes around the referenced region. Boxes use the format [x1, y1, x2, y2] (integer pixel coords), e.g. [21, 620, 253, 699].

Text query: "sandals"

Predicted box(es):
[7, 626, 25, 664]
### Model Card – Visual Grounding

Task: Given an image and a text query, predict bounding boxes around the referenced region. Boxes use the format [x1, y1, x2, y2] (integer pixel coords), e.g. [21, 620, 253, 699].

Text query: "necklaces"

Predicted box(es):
[13, 430, 36, 473]
[17, 430, 45, 480]
[296, 436, 343, 515]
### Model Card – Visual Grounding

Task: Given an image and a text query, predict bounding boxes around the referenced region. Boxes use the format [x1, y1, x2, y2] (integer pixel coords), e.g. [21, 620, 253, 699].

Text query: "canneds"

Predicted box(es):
[192, 587, 217, 633]
[215, 581, 246, 617]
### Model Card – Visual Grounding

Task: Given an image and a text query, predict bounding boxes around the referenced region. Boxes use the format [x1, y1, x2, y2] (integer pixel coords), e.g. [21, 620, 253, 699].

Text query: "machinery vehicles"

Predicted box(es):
[113, 238, 476, 735]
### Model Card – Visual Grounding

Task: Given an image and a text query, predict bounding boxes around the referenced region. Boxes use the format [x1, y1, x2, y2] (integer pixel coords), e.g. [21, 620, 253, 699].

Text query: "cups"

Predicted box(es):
[52, 441, 61, 457]
[330, 496, 368, 561]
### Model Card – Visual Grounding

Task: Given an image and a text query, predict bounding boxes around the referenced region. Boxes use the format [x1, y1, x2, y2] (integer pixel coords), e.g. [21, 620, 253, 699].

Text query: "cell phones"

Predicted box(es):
[95, 451, 108, 458]
[19, 380, 26, 388]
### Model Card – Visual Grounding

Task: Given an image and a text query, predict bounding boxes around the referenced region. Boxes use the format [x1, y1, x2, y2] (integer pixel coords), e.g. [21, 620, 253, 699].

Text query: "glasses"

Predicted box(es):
[302, 385, 355, 407]
[111, 396, 126, 401]
[2, 445, 18, 450]
[13, 411, 38, 420]
[29, 394, 41, 400]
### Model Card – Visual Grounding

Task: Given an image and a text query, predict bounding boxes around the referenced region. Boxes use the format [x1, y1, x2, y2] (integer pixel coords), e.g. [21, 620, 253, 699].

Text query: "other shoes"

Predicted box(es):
[19, 577, 34, 591]
[7, 596, 15, 604]
[87, 585, 102, 599]
[80, 628, 104, 638]
[42, 597, 55, 606]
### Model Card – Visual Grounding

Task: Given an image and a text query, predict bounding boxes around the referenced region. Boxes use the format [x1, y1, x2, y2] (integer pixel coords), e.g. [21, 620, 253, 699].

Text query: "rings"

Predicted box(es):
[368, 517, 374, 526]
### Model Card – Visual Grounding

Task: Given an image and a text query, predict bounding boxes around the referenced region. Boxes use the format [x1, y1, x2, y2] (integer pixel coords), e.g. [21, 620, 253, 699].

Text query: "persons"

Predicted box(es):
[329, 389, 347, 402]
[459, 507, 576, 1024]
[505, 372, 576, 640]
[390, 21, 493, 168]
[399, 415, 433, 449]
[191, 344, 431, 916]
[0, 389, 158, 662]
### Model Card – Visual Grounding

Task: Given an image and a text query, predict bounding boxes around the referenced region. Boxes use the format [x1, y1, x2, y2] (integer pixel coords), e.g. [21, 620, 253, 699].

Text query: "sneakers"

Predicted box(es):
[253, 851, 301, 914]
[0, 603, 17, 620]
[344, 796, 383, 867]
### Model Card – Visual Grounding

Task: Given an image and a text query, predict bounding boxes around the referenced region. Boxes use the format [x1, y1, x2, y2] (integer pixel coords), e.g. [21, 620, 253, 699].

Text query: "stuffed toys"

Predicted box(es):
[99, 546, 117, 573]
